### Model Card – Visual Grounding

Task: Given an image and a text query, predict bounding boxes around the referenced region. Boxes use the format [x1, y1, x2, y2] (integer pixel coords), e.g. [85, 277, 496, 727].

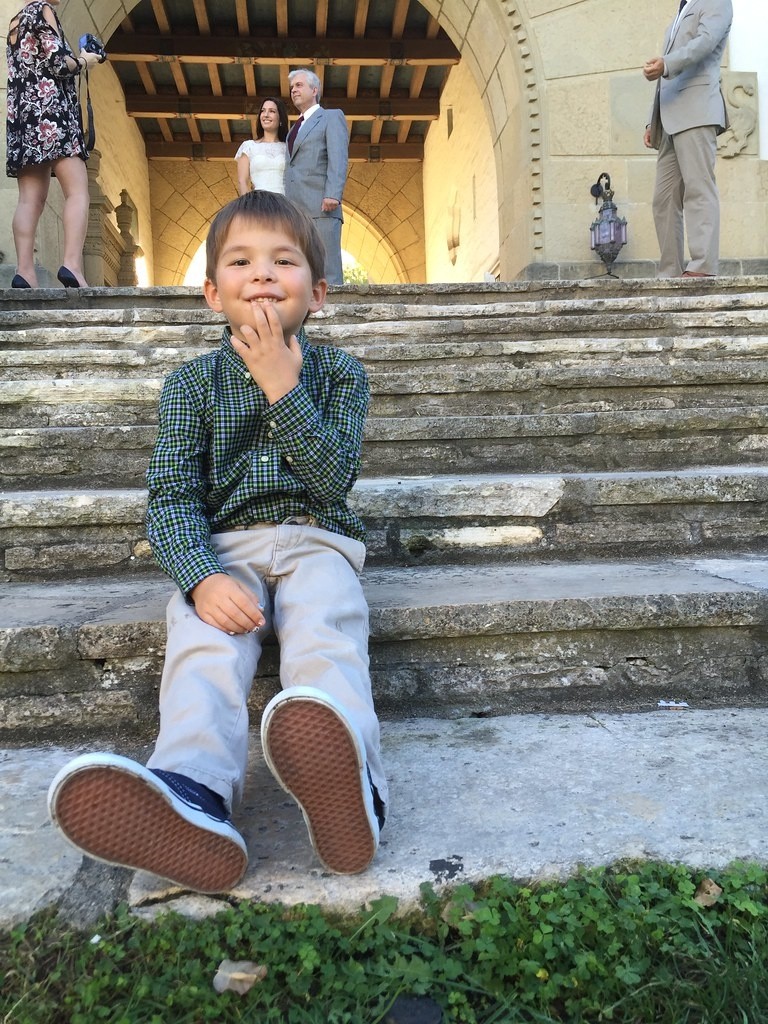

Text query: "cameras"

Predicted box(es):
[79, 33, 106, 64]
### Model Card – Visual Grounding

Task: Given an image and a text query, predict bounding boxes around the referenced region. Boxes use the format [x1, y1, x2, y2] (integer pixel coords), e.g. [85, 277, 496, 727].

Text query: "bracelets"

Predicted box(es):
[79, 56, 87, 70]
[646, 124, 652, 129]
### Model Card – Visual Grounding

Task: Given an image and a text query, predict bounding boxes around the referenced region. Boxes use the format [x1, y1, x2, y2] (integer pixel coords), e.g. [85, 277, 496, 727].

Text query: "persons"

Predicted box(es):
[642, 1, 733, 278]
[183, 97, 288, 287]
[279, 68, 350, 287]
[4, 0, 103, 289]
[49, 187, 389, 895]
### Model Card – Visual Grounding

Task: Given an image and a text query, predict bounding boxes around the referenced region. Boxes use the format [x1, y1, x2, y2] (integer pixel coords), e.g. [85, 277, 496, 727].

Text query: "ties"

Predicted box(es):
[288, 116, 305, 157]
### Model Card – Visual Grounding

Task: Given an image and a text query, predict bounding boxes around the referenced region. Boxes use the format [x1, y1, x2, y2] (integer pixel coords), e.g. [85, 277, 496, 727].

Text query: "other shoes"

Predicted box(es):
[677, 271, 713, 279]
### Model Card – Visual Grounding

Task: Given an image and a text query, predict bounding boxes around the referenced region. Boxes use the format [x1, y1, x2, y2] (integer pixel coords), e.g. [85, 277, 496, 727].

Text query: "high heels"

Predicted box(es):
[11, 274, 32, 288]
[57, 266, 79, 289]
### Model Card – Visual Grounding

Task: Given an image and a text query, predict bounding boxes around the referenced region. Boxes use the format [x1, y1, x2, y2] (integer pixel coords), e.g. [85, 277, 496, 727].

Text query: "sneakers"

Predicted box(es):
[261, 686, 381, 879]
[47, 752, 250, 897]
